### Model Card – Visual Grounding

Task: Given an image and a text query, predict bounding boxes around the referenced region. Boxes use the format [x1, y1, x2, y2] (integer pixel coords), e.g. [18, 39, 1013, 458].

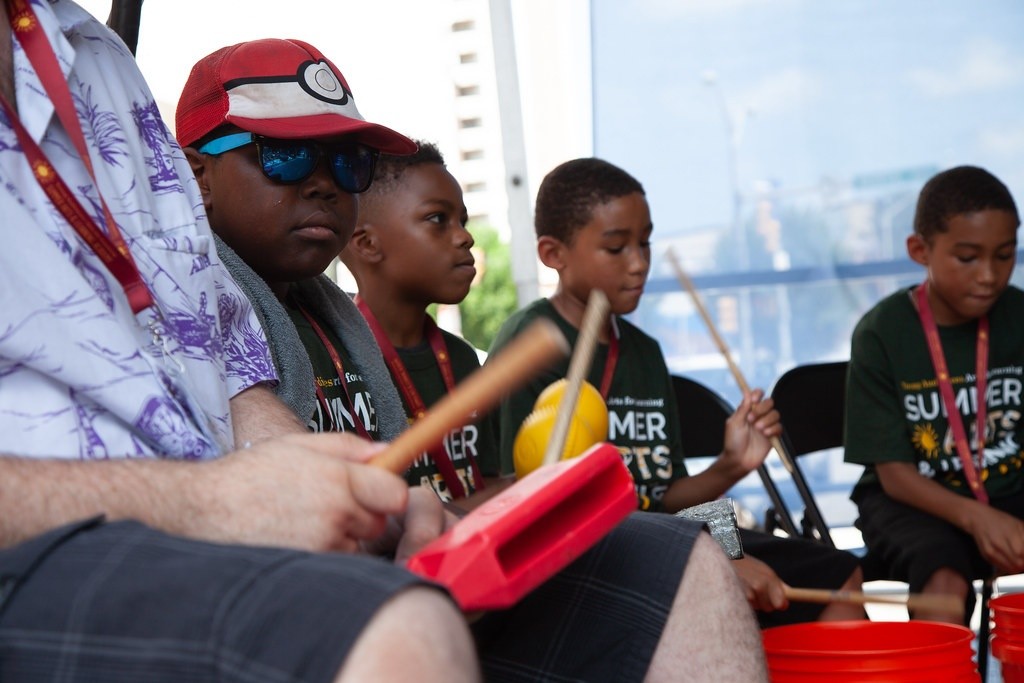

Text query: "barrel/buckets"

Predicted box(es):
[762, 621, 981, 683]
[988, 592, 1024, 683]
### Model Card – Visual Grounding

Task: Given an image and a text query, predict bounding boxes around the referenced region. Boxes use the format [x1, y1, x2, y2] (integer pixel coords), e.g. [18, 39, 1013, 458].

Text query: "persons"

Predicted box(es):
[338, 147, 517, 526]
[175, 38, 479, 569]
[483, 159, 869, 631]
[843, 166, 1024, 630]
[1, 0, 769, 683]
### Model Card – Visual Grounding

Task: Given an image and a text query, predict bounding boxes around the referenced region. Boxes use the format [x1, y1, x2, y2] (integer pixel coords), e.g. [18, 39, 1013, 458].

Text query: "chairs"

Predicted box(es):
[663, 359, 997, 682]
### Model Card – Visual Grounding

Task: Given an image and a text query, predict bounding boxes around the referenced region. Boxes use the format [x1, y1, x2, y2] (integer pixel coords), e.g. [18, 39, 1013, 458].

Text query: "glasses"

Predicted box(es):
[198, 131, 381, 193]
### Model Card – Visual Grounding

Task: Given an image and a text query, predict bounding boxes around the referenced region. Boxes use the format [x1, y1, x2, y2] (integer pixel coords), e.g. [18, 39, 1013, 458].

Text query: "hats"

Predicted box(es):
[175, 38, 419, 156]
